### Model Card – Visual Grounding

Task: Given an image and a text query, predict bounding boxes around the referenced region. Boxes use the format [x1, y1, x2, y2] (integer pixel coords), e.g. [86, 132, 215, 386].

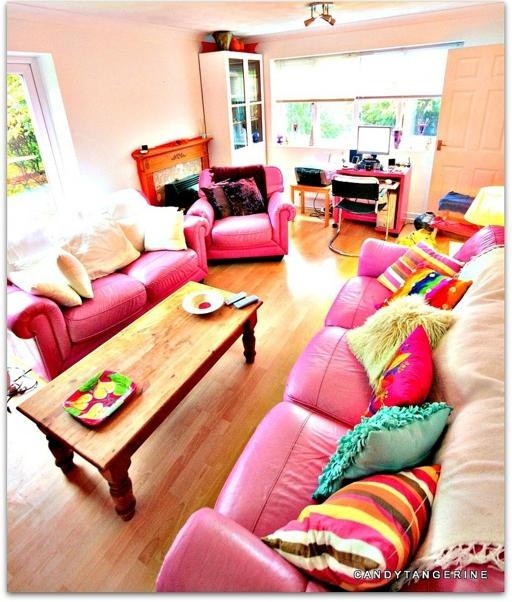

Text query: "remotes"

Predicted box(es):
[233, 295, 258, 310]
[225, 291, 246, 306]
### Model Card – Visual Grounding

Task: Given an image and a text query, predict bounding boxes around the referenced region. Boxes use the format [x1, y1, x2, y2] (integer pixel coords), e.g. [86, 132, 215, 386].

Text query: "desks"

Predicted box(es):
[333, 168, 412, 235]
[290, 185, 333, 228]
[133, 137, 211, 206]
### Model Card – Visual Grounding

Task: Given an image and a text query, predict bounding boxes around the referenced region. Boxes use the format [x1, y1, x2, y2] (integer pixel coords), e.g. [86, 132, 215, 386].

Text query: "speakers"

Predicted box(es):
[389, 159, 395, 166]
[350, 150, 357, 162]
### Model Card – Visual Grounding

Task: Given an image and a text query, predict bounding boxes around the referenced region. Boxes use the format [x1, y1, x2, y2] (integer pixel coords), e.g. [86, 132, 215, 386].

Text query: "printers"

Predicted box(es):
[294, 156, 337, 187]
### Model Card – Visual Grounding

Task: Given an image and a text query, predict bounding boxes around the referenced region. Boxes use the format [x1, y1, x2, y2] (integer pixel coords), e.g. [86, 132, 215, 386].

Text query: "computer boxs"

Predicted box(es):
[377, 191, 397, 229]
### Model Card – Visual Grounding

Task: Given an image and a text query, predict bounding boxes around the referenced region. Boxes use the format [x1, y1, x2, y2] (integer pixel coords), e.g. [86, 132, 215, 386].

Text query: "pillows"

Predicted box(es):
[10, 206, 184, 308]
[261, 463, 441, 591]
[377, 237, 466, 296]
[312, 404, 456, 501]
[365, 326, 433, 417]
[347, 294, 455, 389]
[374, 263, 473, 311]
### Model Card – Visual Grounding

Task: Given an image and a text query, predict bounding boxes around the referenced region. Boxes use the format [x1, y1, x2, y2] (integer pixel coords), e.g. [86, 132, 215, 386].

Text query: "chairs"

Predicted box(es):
[187, 165, 297, 266]
[329, 174, 389, 259]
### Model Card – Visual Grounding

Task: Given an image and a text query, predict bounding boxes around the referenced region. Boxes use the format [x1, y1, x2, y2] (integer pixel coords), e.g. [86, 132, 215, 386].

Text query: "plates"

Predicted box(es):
[181, 289, 225, 315]
[65, 367, 138, 428]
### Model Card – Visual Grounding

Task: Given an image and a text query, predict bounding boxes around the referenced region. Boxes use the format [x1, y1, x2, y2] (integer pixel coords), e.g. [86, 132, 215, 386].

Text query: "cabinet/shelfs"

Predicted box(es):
[198, 51, 267, 168]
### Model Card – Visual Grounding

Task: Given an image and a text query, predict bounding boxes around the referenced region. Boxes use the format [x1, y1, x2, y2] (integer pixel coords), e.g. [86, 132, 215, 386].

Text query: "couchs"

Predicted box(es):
[154, 224, 506, 593]
[7, 188, 211, 381]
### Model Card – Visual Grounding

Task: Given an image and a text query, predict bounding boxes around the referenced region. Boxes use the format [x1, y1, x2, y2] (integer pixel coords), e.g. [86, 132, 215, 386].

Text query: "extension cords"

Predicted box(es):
[321, 206, 332, 215]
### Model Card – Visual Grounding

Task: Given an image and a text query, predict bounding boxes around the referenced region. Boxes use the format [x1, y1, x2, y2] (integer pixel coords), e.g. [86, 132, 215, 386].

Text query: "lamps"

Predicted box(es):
[464, 187, 506, 227]
[304, 2, 339, 27]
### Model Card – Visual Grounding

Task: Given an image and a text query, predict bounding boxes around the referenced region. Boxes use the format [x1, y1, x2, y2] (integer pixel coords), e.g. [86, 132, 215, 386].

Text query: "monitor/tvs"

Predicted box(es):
[357, 126, 391, 163]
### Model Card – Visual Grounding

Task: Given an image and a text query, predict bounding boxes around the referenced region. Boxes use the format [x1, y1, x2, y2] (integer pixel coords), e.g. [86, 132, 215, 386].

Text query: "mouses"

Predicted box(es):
[385, 179, 392, 184]
